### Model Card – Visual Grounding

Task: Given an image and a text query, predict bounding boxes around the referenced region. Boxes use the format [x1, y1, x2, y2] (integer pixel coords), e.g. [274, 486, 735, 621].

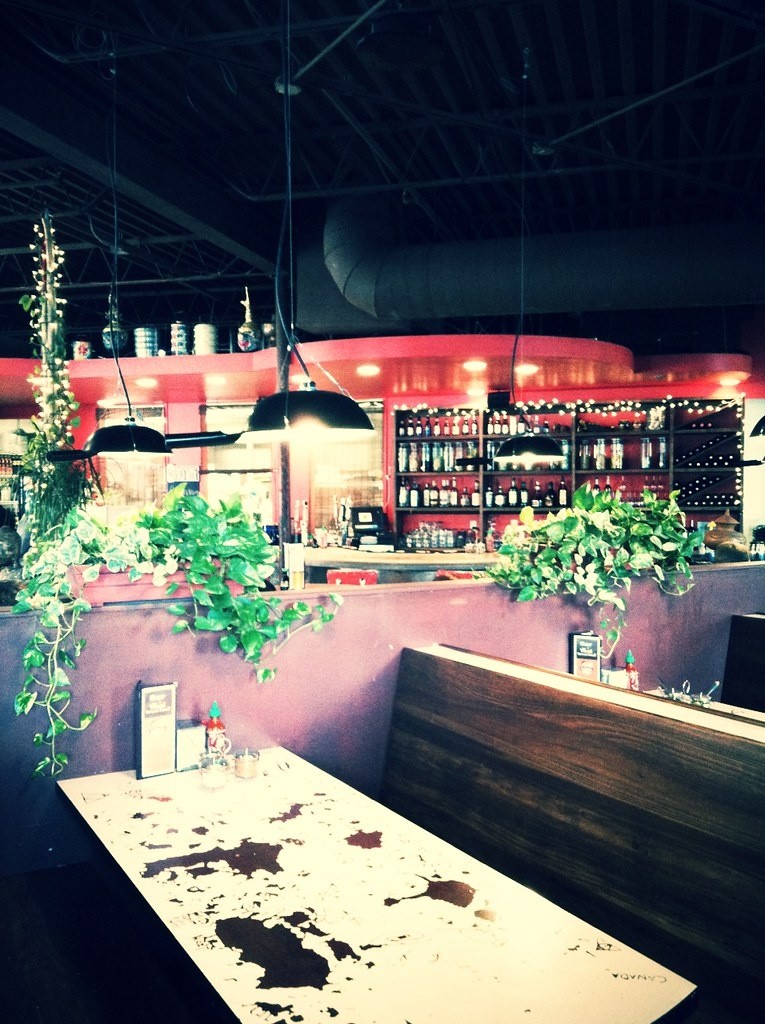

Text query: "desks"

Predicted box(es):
[705, 701, 765, 724]
[55, 745, 697, 1024]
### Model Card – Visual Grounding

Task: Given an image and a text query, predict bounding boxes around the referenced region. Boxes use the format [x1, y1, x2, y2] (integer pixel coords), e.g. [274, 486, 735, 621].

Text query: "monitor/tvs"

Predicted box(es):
[350, 506, 384, 532]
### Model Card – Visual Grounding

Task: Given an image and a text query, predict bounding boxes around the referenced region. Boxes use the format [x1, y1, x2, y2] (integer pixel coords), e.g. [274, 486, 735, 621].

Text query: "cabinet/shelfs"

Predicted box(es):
[382, 396, 747, 556]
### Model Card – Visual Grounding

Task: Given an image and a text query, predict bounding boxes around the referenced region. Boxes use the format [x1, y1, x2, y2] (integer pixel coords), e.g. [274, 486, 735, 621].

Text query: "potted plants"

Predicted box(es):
[18, 484, 350, 778]
[491, 483, 716, 660]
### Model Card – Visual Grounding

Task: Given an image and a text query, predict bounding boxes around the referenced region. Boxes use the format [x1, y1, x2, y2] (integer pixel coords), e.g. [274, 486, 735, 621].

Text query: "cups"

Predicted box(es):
[201, 762, 228, 789]
[72, 340, 93, 360]
[234, 747, 260, 778]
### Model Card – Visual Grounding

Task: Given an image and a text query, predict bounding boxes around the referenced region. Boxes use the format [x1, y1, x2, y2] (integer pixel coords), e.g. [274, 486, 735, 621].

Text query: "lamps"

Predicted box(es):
[41, 0, 377, 463]
[459, 1, 565, 466]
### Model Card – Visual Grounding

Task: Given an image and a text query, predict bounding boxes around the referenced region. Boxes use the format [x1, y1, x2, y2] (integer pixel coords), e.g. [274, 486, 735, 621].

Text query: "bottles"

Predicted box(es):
[387, 404, 695, 555]
[206, 701, 226, 755]
[624, 650, 641, 692]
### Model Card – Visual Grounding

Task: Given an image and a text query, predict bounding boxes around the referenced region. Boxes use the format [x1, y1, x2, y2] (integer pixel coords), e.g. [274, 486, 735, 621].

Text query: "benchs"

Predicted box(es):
[353, 611, 765, 1023]
